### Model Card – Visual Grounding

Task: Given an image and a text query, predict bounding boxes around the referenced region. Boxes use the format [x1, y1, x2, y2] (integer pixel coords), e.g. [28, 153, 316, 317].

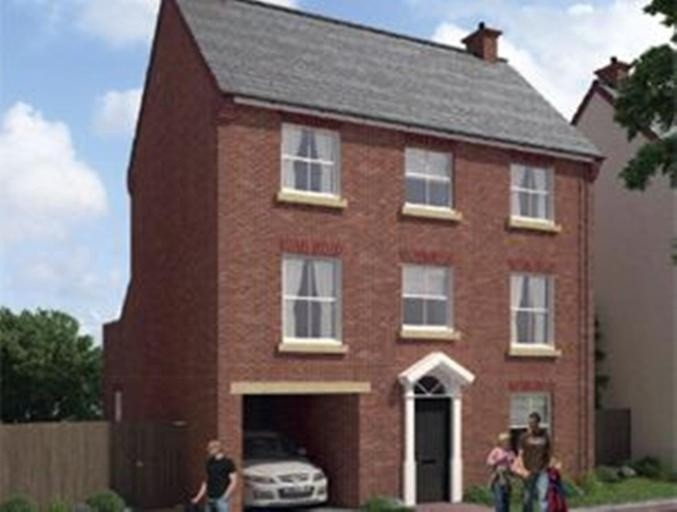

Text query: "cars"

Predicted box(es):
[243, 428, 329, 508]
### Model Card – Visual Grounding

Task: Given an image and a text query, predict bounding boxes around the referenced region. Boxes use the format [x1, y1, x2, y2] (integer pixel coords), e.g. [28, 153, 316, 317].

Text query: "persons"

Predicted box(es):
[485, 432, 516, 512]
[191, 438, 239, 512]
[513, 412, 562, 512]
